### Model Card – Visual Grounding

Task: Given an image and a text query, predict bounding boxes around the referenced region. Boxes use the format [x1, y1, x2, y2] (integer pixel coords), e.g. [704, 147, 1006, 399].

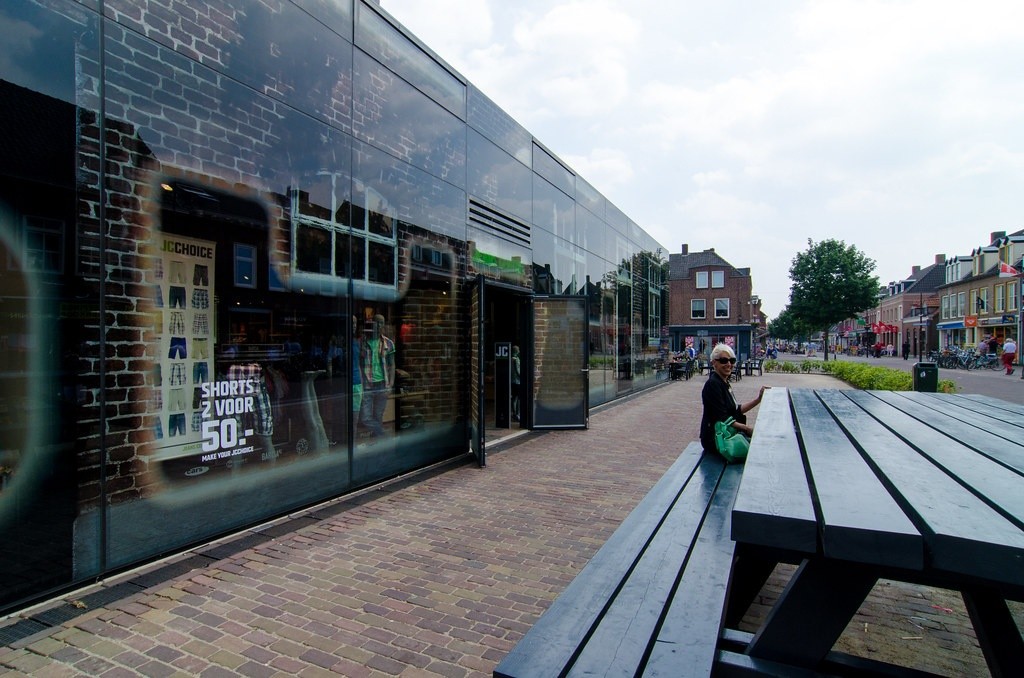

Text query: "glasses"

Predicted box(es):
[714, 357, 736, 364]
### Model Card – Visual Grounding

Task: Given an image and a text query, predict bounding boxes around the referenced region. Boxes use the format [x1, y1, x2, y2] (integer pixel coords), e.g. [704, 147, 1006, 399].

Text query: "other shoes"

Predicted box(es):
[1010, 368, 1015, 375]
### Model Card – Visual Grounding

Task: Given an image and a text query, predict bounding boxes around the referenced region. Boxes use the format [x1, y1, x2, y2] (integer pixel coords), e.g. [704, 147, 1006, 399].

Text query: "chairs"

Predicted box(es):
[731, 358, 763, 382]
[669, 360, 694, 381]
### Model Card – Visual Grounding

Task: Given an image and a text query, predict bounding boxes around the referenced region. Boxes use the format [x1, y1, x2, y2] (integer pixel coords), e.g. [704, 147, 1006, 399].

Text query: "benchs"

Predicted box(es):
[491, 439, 747, 678]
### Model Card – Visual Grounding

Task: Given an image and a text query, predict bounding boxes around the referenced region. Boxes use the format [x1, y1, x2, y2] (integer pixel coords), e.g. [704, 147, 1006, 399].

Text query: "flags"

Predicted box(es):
[998, 261, 1017, 277]
[977, 297, 985, 310]
[871, 321, 890, 334]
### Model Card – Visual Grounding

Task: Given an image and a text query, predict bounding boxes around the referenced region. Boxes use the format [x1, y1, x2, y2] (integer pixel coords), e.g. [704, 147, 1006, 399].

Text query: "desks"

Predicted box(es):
[730, 388, 1024, 678]
[743, 362, 758, 375]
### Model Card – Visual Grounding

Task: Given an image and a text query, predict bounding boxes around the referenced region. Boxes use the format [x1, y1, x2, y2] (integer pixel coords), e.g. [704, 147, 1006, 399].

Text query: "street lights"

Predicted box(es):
[863, 323, 871, 358]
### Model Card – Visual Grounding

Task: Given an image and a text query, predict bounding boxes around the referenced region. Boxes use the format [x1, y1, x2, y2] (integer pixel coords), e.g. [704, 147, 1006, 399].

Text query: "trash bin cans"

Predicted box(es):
[912, 362, 938, 393]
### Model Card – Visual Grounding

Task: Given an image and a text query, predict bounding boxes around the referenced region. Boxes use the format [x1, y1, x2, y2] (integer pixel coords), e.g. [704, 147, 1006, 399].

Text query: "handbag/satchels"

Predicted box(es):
[715, 416, 750, 464]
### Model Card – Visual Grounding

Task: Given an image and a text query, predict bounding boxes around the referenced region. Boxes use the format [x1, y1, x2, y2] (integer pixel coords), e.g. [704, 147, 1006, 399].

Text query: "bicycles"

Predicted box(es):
[926, 344, 1006, 372]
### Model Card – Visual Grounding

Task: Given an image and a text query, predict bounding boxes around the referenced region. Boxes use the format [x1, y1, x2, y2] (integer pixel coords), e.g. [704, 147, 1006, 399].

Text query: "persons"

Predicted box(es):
[325, 314, 395, 443]
[874, 341, 892, 358]
[511, 346, 521, 422]
[979, 338, 999, 368]
[300, 370, 329, 455]
[227, 365, 277, 473]
[901, 340, 911, 361]
[697, 342, 771, 462]
[766, 343, 777, 360]
[1003, 338, 1017, 375]
